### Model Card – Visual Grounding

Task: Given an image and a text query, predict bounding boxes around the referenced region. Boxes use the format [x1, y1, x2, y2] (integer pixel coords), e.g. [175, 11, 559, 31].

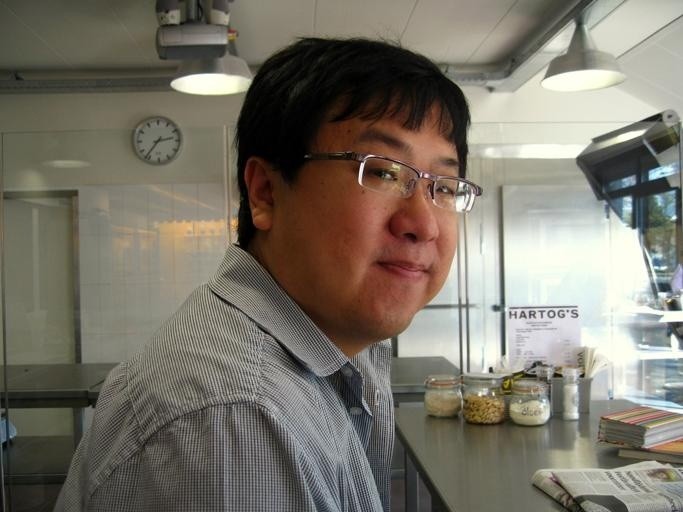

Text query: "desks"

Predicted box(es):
[86, 354, 465, 511]
[394, 398, 683, 511]
[0, 363, 118, 512]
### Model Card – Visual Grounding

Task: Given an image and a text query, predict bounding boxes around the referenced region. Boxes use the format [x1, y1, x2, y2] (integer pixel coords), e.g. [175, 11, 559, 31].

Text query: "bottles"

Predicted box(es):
[423, 373, 463, 419]
[460, 372, 507, 425]
[560, 367, 581, 423]
[509, 376, 551, 427]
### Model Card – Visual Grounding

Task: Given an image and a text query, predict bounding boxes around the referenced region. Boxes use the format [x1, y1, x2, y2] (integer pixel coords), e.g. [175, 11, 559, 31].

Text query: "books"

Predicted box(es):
[618, 440, 683, 464]
[597, 405, 683, 449]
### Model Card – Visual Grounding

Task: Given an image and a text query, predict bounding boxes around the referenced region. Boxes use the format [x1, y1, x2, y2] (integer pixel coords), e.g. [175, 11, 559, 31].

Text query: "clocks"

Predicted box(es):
[130, 116, 184, 166]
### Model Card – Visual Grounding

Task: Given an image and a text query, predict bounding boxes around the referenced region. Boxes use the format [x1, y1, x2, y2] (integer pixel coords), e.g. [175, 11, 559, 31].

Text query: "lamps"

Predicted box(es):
[169, 31, 254, 100]
[539, 11, 628, 97]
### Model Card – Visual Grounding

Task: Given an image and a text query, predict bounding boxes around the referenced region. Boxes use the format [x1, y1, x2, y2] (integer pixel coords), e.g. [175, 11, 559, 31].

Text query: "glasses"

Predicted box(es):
[299, 151, 482, 213]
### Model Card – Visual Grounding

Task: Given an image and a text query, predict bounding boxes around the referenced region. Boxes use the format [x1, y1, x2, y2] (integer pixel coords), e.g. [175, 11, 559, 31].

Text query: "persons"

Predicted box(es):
[52, 36, 463, 512]
